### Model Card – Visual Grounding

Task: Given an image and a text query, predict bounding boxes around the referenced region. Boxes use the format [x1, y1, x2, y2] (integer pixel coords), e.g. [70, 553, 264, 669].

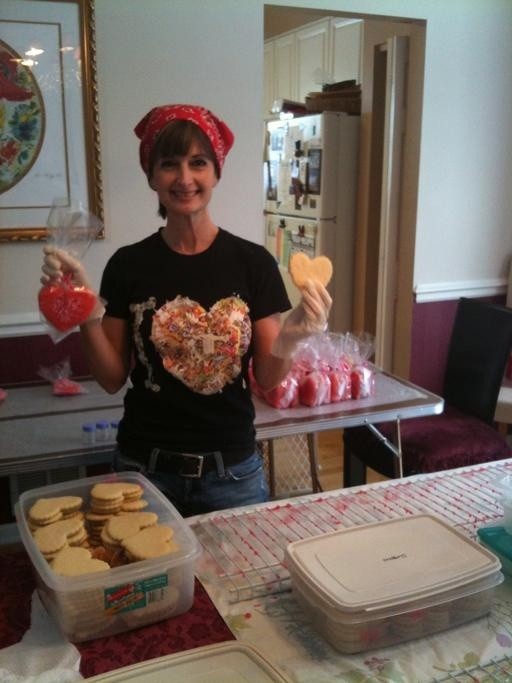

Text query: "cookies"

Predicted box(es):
[28, 484, 180, 637]
[289, 253, 332, 290]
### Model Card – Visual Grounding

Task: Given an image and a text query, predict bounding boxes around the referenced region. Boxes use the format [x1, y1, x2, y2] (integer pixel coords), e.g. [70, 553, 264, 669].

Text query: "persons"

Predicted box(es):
[40, 103, 333, 520]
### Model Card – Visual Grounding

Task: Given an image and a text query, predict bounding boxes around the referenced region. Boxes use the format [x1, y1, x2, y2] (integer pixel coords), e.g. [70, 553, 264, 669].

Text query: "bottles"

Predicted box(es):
[95, 420, 111, 442]
[81, 422, 96, 444]
[110, 420, 121, 442]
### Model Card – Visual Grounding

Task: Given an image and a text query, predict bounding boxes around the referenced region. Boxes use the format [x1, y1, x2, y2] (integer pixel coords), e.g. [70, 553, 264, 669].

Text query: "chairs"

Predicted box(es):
[343, 297, 512, 488]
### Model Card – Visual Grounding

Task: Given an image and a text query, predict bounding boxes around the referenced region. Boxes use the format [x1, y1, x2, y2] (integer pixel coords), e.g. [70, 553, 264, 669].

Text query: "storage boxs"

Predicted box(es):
[15, 471, 201, 644]
[286, 512, 504, 654]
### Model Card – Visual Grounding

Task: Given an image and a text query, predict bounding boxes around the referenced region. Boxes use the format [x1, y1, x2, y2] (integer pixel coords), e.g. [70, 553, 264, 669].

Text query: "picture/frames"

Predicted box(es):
[0, 0, 104, 242]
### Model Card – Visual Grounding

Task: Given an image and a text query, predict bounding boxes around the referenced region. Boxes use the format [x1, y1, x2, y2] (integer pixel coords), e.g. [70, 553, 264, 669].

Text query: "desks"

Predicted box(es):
[0, 456, 512, 683]
[0, 360, 445, 499]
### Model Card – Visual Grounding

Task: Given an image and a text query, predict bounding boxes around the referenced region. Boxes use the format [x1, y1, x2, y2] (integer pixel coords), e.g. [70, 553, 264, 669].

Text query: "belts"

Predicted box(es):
[109, 442, 259, 477]
